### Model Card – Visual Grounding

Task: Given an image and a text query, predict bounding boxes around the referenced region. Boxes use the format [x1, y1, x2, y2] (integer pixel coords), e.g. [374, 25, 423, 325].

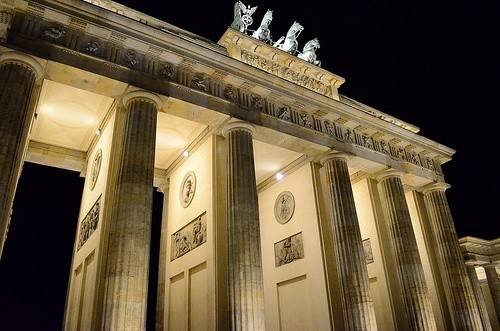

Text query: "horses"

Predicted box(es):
[229, 2, 245, 33]
[277, 21, 305, 54]
[297, 37, 322, 68]
[251, 9, 273, 45]
